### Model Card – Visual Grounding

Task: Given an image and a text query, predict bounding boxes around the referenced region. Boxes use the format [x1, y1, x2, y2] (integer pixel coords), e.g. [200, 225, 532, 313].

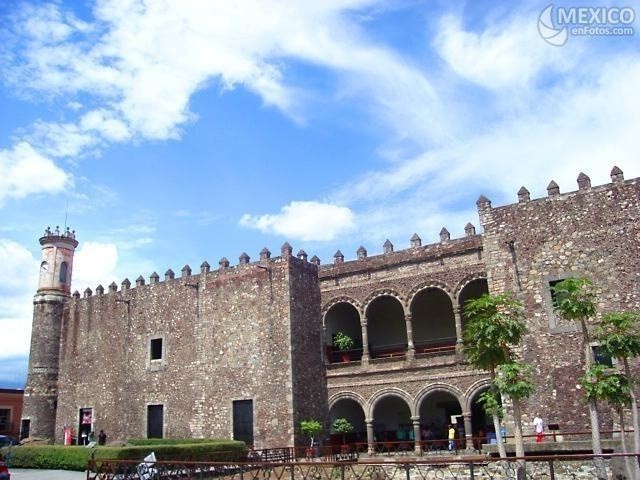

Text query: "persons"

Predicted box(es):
[532, 412, 545, 444]
[88, 431, 97, 445]
[98, 429, 106, 446]
[396, 416, 485, 453]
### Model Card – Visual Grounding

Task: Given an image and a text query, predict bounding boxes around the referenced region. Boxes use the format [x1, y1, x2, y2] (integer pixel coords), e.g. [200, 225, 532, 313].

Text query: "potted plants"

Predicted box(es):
[331, 417, 354, 454]
[334, 332, 355, 365]
[300, 419, 323, 459]
